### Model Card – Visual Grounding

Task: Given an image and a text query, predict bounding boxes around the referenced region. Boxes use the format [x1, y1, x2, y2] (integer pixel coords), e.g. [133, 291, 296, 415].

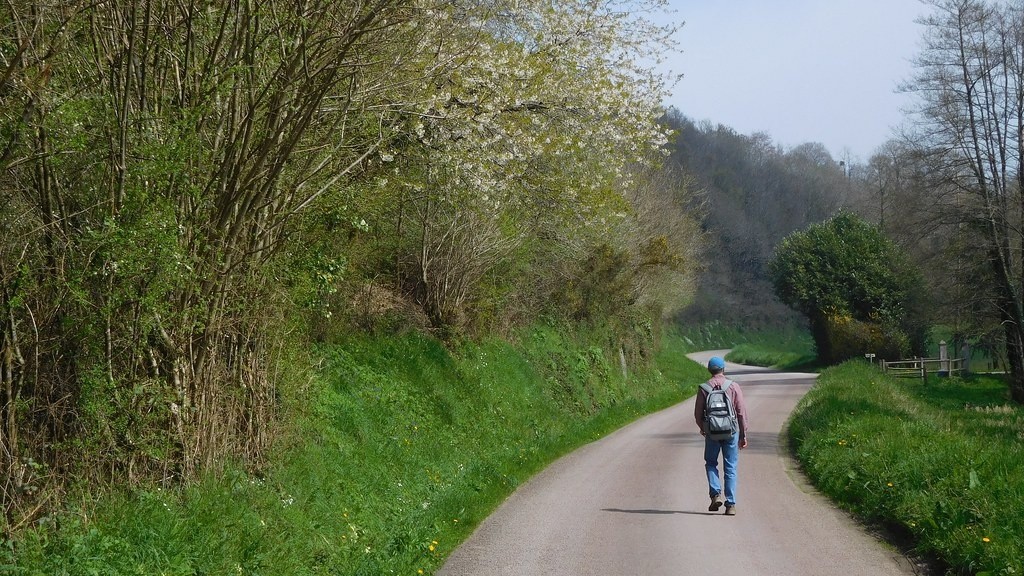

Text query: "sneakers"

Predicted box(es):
[725, 505, 736, 514]
[709, 492, 723, 511]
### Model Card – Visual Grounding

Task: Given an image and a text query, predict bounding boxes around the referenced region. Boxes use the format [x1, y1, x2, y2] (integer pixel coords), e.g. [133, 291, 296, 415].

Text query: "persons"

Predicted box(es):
[693, 356, 747, 515]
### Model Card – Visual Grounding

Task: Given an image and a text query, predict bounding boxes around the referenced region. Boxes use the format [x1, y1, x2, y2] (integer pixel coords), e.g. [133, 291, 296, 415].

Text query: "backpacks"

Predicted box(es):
[698, 380, 737, 440]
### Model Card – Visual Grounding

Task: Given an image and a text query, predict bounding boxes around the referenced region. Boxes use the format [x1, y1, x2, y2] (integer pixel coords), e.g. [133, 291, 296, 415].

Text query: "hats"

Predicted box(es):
[708, 357, 724, 370]
